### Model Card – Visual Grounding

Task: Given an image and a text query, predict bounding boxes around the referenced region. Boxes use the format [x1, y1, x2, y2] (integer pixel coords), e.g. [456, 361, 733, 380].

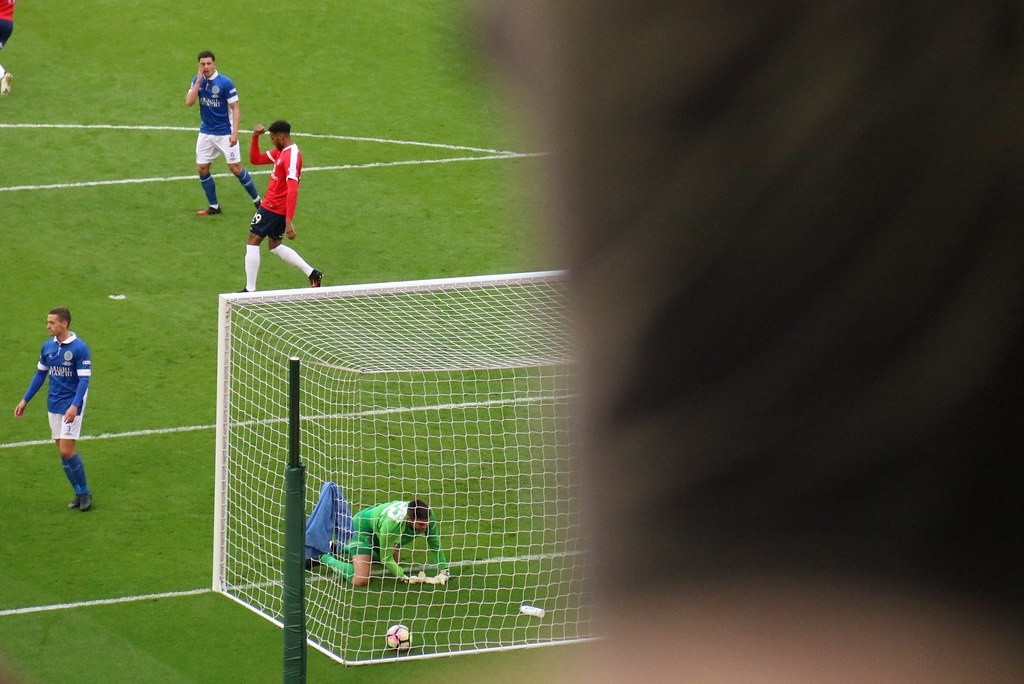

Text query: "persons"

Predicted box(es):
[305, 499, 448, 585]
[452, 1, 1023, 684]
[14, 308, 93, 512]
[184, 51, 263, 216]
[0, 0, 15, 93]
[240, 121, 322, 293]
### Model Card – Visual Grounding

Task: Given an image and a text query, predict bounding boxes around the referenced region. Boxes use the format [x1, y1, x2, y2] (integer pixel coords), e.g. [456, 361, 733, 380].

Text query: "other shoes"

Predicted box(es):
[329, 541, 339, 553]
[306, 556, 321, 569]
[1, 73, 13, 95]
[67, 496, 79, 508]
[80, 494, 92, 511]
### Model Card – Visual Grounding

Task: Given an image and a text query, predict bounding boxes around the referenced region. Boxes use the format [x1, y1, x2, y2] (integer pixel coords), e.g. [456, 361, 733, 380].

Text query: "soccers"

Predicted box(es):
[385, 624, 413, 652]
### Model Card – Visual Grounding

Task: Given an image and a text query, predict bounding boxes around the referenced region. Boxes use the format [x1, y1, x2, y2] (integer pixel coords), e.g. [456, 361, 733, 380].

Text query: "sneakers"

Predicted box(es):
[197, 204, 221, 215]
[309, 268, 322, 287]
[239, 288, 247, 292]
[254, 199, 262, 209]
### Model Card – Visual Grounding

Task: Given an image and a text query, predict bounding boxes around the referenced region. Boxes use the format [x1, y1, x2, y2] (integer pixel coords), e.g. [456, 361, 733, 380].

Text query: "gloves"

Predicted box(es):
[408, 571, 426, 583]
[426, 569, 449, 585]
